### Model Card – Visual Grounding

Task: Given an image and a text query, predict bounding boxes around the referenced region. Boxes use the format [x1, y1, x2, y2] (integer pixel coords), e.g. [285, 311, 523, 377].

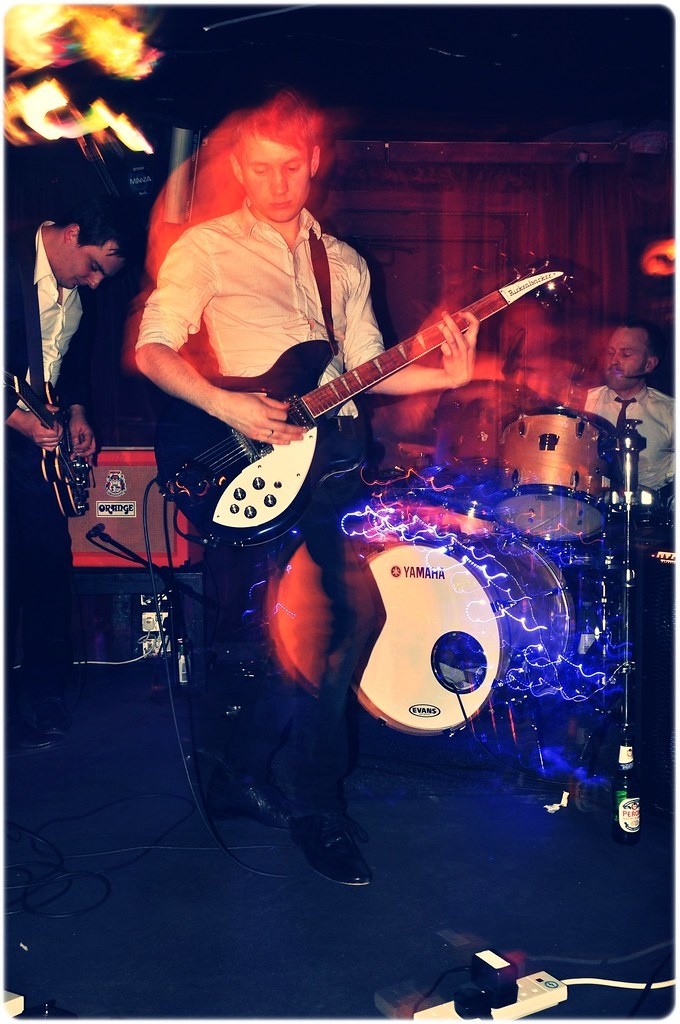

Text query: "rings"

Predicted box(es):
[268, 430, 274, 439]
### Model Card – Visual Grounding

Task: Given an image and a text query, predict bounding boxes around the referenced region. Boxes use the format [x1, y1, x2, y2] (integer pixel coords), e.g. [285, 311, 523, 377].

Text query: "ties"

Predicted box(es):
[614, 397, 636, 473]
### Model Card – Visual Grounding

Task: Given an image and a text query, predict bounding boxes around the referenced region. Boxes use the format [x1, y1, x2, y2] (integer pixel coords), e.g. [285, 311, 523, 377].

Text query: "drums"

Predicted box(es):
[259, 378, 630, 739]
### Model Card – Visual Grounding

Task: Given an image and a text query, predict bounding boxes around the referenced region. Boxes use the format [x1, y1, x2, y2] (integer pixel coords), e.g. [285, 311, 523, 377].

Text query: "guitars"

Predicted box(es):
[4, 364, 95, 515]
[155, 257, 584, 547]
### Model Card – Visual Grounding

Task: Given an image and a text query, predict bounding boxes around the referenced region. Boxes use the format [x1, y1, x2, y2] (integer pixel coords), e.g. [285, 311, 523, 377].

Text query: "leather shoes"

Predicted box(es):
[8, 716, 69, 757]
[201, 777, 296, 831]
[290, 801, 373, 887]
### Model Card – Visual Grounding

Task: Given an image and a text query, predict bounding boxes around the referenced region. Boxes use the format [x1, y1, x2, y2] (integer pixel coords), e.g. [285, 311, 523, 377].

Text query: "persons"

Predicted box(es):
[585, 324, 674, 492]
[5, 202, 127, 747]
[136, 111, 479, 885]
